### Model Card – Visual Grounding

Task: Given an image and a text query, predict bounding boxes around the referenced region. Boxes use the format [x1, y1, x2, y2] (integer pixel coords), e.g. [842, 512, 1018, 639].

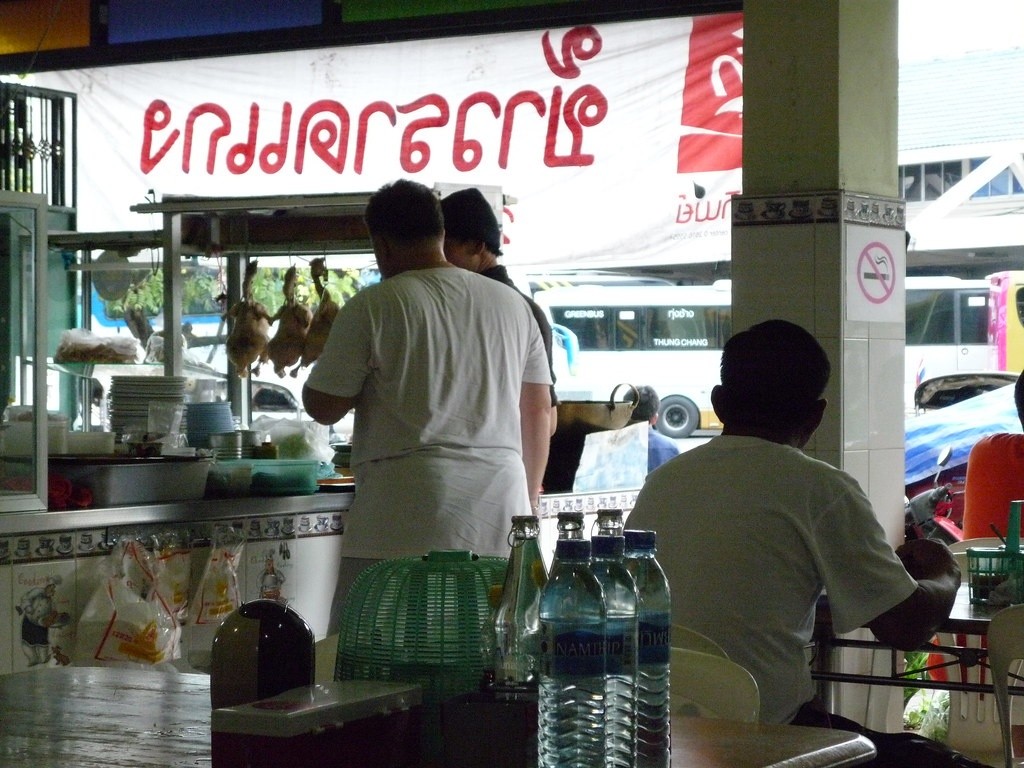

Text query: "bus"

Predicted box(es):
[503, 267, 1024, 545]
[73, 257, 356, 468]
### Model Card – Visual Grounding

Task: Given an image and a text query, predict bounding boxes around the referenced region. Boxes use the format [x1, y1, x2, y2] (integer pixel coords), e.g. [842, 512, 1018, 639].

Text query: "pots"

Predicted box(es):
[554, 382, 640, 439]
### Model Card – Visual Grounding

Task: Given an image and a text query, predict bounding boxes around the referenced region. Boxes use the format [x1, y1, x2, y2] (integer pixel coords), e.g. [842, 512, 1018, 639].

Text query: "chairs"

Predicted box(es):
[528, 534, 1024, 768]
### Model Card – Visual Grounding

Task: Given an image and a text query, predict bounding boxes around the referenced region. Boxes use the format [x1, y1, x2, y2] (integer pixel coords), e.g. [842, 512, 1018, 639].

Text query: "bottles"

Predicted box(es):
[537, 539, 607, 768]
[494, 514, 548, 690]
[591, 534, 639, 768]
[621, 527, 672, 768]
[597, 507, 625, 536]
[549, 511, 585, 581]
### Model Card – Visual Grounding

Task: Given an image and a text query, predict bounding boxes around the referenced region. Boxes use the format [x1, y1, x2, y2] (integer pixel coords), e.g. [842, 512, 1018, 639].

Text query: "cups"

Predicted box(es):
[207, 429, 262, 460]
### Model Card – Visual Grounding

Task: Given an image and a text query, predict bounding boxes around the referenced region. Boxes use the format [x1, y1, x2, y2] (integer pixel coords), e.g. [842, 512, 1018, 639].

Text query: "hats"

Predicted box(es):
[442, 188, 501, 248]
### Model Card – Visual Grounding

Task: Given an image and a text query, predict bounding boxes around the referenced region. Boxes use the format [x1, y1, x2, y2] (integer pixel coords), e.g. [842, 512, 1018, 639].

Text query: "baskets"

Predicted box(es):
[966, 501, 1024, 607]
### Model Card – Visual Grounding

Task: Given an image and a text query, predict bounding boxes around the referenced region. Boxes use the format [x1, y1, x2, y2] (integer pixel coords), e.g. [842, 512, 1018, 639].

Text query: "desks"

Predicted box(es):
[0, 667, 878, 768]
[806, 582, 1024, 697]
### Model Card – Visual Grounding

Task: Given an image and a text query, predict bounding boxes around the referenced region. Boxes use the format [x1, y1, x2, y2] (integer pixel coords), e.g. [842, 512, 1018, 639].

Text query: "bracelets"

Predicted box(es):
[532, 495, 542, 512]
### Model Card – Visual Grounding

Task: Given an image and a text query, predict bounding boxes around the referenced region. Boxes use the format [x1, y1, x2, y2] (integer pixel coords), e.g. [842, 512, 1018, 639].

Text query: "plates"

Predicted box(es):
[109, 375, 188, 440]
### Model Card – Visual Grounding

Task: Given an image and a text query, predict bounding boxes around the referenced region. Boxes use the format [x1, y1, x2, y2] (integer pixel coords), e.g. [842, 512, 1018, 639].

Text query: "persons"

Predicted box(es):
[624, 385, 680, 474]
[302, 178, 555, 634]
[625, 319, 990, 768]
[440, 187, 561, 436]
[928, 368, 1024, 680]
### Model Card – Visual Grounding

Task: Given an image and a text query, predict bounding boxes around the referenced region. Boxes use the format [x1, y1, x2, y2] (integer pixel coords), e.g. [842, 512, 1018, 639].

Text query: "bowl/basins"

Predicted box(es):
[205, 461, 254, 498]
[2, 421, 68, 456]
[67, 430, 116, 454]
